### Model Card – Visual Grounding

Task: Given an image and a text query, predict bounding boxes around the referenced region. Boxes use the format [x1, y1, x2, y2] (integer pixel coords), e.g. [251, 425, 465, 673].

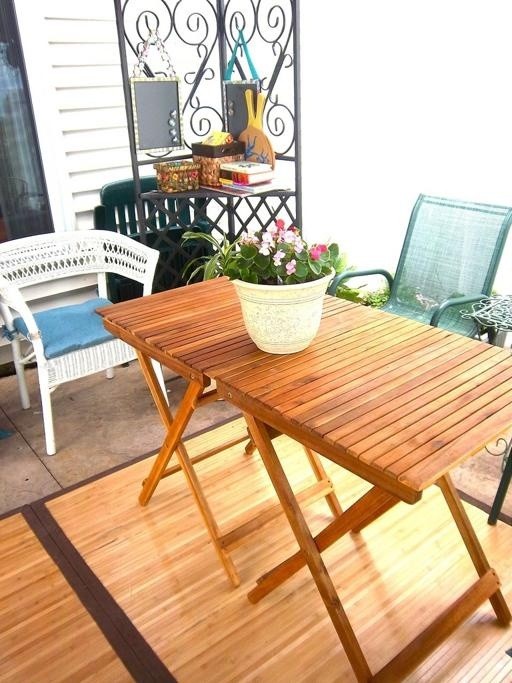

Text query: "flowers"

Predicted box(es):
[225, 219, 345, 285]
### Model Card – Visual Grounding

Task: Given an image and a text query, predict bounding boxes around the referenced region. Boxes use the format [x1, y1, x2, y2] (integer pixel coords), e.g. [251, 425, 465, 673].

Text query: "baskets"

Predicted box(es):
[153, 162, 201, 193]
[192, 140, 246, 187]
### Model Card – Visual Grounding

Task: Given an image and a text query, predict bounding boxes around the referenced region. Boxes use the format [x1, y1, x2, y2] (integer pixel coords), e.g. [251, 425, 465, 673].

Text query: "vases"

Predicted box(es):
[228, 268, 337, 355]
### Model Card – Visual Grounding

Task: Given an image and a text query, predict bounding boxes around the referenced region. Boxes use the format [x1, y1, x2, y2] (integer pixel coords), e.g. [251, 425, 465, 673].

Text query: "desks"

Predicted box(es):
[96, 272, 511, 682]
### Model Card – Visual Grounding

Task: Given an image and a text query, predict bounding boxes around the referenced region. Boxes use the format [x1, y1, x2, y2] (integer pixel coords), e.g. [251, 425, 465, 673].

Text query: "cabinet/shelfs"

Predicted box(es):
[113, 0, 302, 295]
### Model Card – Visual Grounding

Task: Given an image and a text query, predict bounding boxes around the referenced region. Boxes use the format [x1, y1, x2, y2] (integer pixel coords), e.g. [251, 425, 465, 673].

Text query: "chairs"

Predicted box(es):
[94, 176, 214, 368]
[329, 193, 512, 339]
[0, 228, 171, 456]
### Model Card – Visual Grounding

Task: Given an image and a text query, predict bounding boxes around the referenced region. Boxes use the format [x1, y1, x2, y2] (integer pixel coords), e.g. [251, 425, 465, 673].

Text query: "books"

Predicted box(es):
[197, 159, 291, 199]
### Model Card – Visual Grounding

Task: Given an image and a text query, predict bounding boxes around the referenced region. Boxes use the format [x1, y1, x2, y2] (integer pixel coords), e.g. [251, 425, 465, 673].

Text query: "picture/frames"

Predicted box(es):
[220, 80, 263, 141]
[131, 78, 185, 153]
